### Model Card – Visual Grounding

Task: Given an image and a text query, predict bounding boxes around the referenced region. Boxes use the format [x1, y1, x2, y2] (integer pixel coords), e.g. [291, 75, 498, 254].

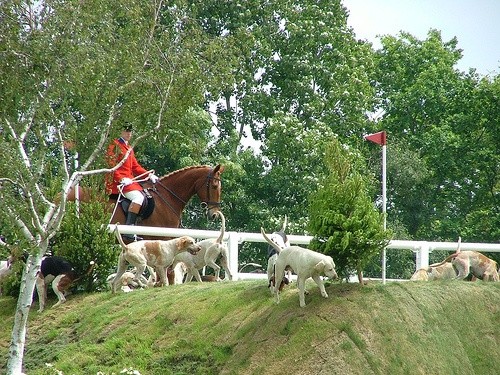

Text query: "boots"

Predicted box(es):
[121, 211, 144, 241]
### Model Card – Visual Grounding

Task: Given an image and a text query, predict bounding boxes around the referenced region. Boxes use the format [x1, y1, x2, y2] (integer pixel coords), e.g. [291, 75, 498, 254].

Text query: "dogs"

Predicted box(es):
[261, 214, 338, 309]
[409, 236, 500, 283]
[0, 255, 95, 313]
[105, 209, 232, 294]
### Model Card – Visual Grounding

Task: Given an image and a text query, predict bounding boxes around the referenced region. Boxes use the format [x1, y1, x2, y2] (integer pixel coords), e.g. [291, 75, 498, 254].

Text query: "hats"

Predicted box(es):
[121, 122, 134, 132]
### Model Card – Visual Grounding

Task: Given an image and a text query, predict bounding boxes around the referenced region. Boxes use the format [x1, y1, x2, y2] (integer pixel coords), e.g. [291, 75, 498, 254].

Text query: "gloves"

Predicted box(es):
[118, 177, 133, 185]
[148, 172, 158, 184]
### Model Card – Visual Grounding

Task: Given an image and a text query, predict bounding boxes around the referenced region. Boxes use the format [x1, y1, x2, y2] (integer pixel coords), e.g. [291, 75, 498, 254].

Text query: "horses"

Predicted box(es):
[64, 164, 224, 246]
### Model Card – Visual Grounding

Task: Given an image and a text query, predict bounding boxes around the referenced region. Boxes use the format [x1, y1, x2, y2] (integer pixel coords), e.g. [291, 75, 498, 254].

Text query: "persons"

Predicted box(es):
[99, 122, 158, 241]
[44, 137, 83, 202]
